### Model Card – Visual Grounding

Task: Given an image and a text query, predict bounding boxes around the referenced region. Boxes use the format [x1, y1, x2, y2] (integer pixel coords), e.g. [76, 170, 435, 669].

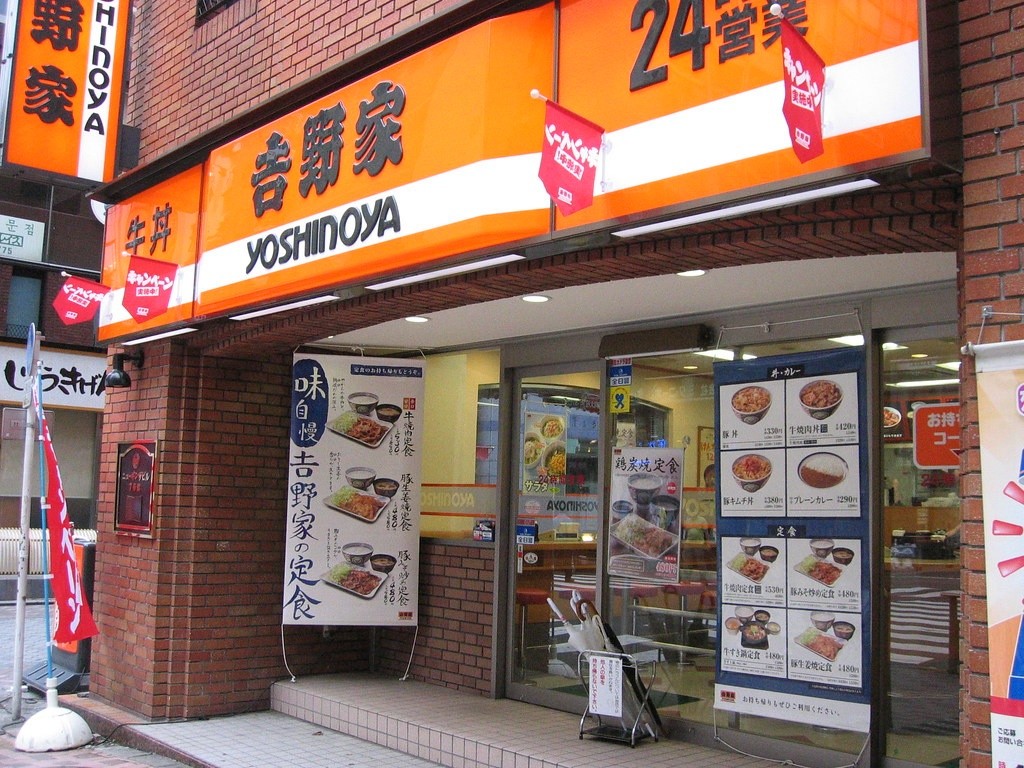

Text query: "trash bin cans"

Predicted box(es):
[23, 537, 96, 696]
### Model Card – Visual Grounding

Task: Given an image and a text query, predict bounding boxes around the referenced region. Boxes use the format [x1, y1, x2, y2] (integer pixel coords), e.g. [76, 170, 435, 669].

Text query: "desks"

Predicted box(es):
[516, 540, 718, 623]
[882, 548, 961, 691]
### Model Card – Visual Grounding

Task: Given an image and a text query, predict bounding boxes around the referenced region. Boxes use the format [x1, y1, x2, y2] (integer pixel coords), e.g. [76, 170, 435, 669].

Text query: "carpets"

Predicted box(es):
[548, 684, 704, 710]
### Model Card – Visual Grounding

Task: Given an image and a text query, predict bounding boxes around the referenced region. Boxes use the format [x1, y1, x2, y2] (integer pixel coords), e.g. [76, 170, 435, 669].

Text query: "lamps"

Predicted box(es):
[105, 349, 145, 388]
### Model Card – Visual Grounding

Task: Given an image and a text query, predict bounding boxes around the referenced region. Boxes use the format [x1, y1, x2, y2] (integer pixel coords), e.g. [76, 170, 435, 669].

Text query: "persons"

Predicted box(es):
[681, 511, 708, 587]
[554, 508, 574, 582]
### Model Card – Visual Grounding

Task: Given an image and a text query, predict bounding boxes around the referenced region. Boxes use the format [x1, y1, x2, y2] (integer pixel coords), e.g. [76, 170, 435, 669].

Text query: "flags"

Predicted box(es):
[32, 374, 100, 645]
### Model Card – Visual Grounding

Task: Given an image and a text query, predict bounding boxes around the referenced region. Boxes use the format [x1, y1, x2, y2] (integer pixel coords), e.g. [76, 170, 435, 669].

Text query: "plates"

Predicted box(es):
[610, 513, 679, 562]
[741, 621, 769, 650]
[794, 626, 845, 662]
[703, 463, 719, 489]
[320, 561, 388, 599]
[724, 552, 770, 583]
[323, 485, 392, 523]
[326, 410, 394, 449]
[793, 552, 848, 589]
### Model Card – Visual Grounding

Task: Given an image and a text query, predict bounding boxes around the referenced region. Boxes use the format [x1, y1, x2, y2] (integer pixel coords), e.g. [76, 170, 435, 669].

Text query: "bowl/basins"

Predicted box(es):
[345, 467, 376, 490]
[613, 472, 679, 525]
[809, 611, 856, 640]
[884, 407, 902, 431]
[809, 537, 854, 567]
[370, 553, 397, 575]
[341, 543, 374, 566]
[740, 537, 779, 563]
[730, 385, 772, 425]
[798, 380, 844, 421]
[524, 415, 565, 479]
[348, 391, 380, 415]
[797, 452, 849, 489]
[376, 404, 403, 423]
[725, 605, 781, 637]
[373, 478, 399, 497]
[731, 454, 772, 493]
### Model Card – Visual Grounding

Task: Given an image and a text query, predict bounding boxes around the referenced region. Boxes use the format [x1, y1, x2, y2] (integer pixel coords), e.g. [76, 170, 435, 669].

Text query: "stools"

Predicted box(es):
[515, 579, 718, 691]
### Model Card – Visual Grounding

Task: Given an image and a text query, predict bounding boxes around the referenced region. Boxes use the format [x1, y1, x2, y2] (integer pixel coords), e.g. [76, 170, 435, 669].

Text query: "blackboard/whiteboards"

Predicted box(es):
[587, 655, 622, 717]
